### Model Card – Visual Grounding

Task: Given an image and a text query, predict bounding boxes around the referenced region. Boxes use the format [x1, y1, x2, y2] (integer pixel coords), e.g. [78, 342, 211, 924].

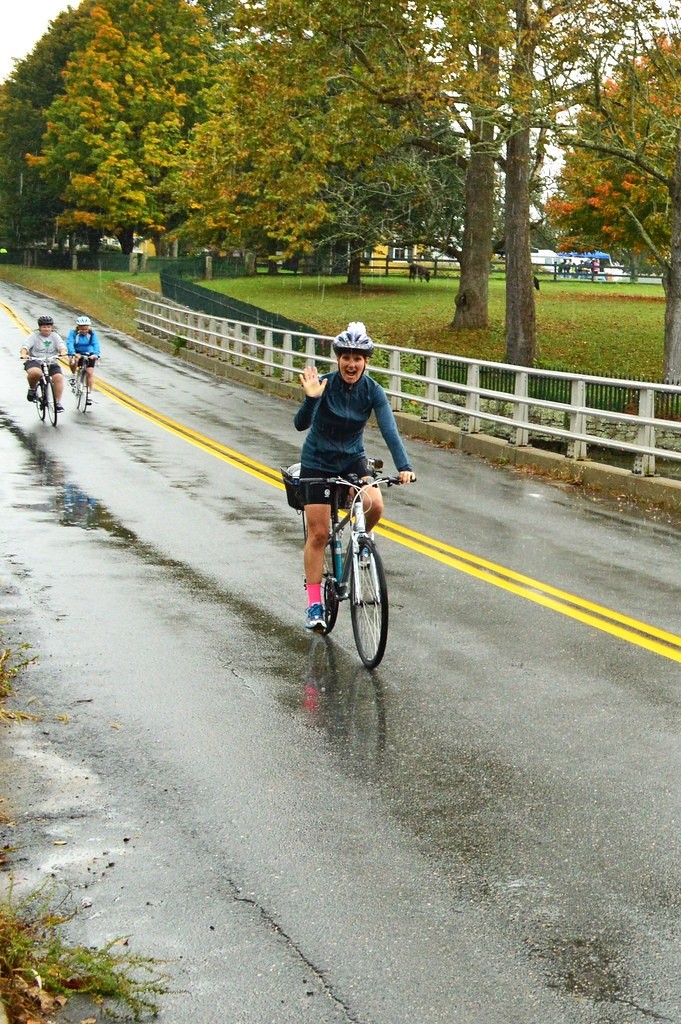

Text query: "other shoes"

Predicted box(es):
[69, 378, 76, 385]
[87, 398, 92, 405]
[27, 388, 38, 403]
[56, 403, 64, 412]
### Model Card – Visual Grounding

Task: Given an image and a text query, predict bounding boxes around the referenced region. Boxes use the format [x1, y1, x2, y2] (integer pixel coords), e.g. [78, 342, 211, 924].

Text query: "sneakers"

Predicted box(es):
[304, 603, 328, 630]
[359, 531, 374, 568]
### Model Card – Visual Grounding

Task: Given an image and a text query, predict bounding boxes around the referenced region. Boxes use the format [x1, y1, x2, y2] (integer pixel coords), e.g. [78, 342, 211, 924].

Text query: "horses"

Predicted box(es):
[409, 263, 430, 283]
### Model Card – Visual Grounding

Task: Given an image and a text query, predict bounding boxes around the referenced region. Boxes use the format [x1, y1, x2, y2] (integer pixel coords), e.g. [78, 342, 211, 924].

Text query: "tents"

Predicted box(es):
[556, 250, 613, 265]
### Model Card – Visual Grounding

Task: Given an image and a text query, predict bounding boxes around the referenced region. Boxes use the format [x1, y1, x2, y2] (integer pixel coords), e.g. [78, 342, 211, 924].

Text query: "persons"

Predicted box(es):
[560, 259, 599, 277]
[294, 322, 414, 628]
[66, 316, 101, 405]
[19, 316, 67, 413]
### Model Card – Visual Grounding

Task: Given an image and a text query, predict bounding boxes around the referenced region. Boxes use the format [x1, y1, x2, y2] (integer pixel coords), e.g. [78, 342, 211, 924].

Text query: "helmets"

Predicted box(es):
[333, 328, 373, 358]
[76, 315, 91, 326]
[38, 316, 55, 325]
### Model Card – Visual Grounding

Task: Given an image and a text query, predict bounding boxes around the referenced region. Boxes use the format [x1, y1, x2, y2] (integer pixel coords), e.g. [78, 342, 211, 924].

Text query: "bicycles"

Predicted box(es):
[279, 457, 417, 668]
[69, 355, 100, 413]
[20, 354, 67, 426]
